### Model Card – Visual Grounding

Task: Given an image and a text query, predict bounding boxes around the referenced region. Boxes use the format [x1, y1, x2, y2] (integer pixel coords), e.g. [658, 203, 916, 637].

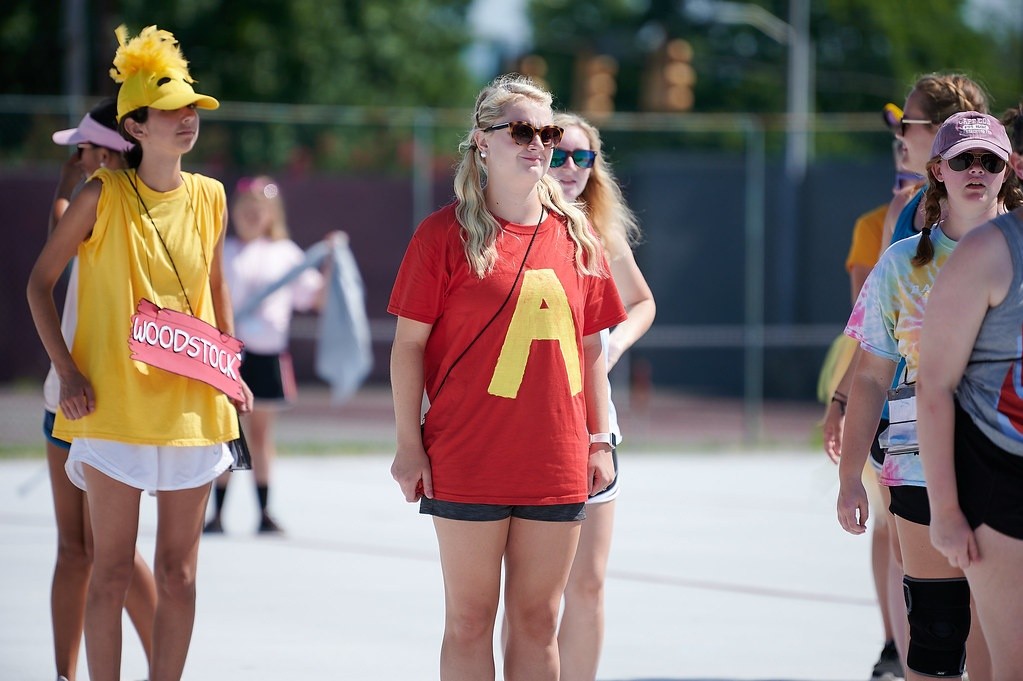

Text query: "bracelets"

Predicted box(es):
[835, 390, 848, 399]
[831, 396, 847, 416]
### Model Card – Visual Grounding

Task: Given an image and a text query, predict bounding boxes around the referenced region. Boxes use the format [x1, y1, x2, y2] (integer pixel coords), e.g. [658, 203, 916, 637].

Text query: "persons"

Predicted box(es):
[27, 22, 254, 681]
[821, 74, 1023, 681]
[199, 175, 350, 536]
[386, 76, 656, 680]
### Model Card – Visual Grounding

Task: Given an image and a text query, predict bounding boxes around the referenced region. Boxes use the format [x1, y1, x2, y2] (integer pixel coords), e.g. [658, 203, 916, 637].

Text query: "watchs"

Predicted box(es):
[588, 432, 617, 450]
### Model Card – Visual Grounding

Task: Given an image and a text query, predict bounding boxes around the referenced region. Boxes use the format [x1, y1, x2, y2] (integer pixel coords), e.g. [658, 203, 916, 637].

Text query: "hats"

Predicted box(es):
[110, 25, 220, 124]
[931, 111, 1012, 160]
[52, 113, 136, 153]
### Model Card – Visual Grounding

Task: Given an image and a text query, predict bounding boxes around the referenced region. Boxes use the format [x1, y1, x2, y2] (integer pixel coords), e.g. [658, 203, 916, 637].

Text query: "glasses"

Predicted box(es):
[894, 172, 925, 190]
[484, 120, 564, 149]
[940, 152, 1006, 174]
[549, 148, 597, 169]
[76, 143, 93, 153]
[900, 117, 933, 137]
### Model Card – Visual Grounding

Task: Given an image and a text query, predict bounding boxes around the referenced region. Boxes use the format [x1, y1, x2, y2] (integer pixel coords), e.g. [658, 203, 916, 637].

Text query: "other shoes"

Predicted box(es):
[258, 518, 278, 531]
[203, 519, 223, 533]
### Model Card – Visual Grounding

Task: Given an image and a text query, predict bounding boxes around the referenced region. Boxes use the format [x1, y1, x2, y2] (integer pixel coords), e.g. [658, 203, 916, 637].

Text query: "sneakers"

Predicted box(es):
[871, 641, 905, 681]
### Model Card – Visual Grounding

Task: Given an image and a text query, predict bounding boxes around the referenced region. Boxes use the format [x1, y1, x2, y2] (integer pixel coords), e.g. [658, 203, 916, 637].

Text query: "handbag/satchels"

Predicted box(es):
[228, 408, 252, 470]
[887, 385, 919, 455]
